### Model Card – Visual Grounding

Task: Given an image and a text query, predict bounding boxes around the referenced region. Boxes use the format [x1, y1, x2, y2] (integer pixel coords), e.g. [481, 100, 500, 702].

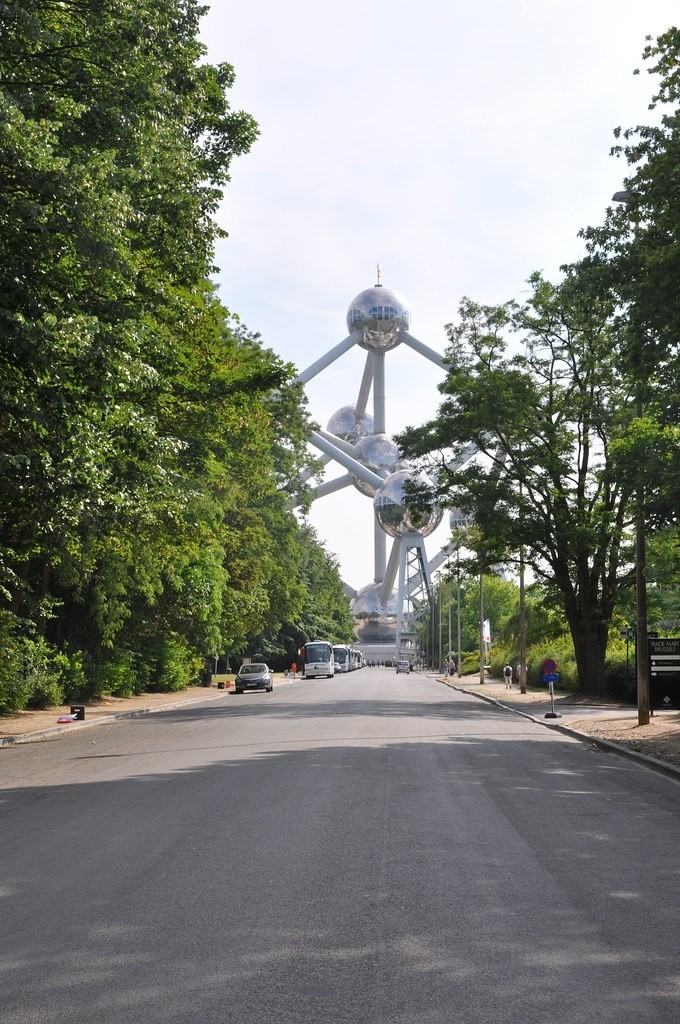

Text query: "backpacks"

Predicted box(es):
[505, 666, 510, 677]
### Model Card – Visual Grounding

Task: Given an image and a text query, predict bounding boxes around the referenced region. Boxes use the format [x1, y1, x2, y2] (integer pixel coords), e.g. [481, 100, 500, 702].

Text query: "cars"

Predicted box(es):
[235, 663, 274, 695]
[396, 661, 410, 674]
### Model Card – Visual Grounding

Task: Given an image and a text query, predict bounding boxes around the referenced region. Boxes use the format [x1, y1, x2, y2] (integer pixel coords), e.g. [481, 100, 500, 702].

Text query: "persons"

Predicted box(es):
[449, 660, 456, 677]
[442, 659, 449, 679]
[516, 661, 528, 689]
[362, 660, 384, 668]
[291, 661, 297, 679]
[502, 662, 513, 689]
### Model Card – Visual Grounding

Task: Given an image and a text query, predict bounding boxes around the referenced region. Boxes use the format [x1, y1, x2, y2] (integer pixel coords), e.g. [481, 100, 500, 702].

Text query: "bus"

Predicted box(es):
[301, 639, 364, 679]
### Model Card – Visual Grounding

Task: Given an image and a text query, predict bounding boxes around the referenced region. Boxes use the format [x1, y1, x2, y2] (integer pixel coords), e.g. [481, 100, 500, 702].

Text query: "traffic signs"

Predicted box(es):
[647, 639, 680, 679]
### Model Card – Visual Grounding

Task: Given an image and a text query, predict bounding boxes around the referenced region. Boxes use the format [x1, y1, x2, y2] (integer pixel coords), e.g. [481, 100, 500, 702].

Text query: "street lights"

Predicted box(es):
[442, 554, 452, 675]
[510, 478, 528, 694]
[447, 544, 461, 677]
[426, 582, 435, 671]
